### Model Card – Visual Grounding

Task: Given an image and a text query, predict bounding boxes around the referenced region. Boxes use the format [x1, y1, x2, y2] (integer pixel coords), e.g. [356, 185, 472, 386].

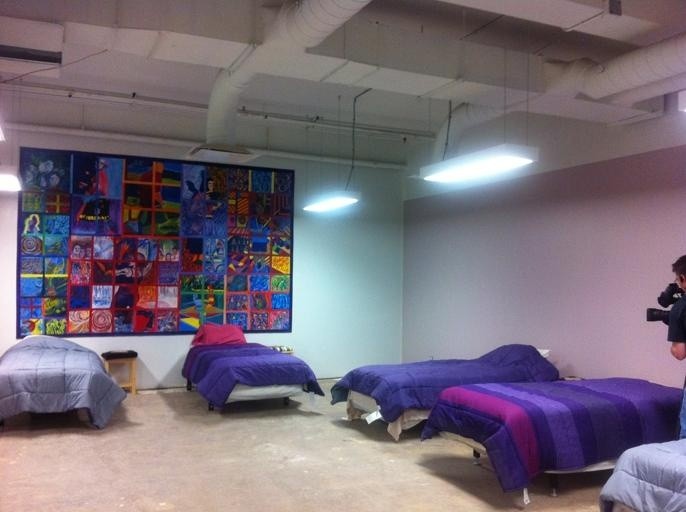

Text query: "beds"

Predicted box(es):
[0, 334, 126, 434]
[598, 434, 686, 512]
[328, 338, 560, 443]
[181, 318, 329, 414]
[418, 371, 686, 505]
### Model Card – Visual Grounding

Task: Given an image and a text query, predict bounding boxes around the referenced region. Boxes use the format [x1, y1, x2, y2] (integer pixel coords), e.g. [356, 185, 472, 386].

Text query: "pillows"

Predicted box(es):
[195, 319, 245, 343]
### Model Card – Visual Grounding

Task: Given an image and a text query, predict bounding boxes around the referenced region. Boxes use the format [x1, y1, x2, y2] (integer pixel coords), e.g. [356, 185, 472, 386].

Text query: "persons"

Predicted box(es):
[667, 255, 686, 442]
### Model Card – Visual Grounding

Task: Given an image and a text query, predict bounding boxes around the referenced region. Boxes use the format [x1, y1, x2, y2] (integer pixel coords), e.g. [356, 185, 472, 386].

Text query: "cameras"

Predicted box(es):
[647, 283, 678, 324]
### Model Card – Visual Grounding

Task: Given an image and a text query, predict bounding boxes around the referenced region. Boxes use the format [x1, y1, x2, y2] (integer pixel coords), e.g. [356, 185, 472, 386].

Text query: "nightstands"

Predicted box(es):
[261, 345, 294, 356]
[101, 349, 139, 399]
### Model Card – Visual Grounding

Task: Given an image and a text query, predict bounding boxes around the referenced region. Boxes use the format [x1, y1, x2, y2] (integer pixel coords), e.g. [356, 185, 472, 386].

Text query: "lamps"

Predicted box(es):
[419, 46, 544, 186]
[300, 93, 363, 216]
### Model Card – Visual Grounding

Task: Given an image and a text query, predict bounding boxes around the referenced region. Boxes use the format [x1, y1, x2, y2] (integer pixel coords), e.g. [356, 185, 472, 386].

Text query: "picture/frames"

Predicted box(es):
[13, 143, 297, 339]
